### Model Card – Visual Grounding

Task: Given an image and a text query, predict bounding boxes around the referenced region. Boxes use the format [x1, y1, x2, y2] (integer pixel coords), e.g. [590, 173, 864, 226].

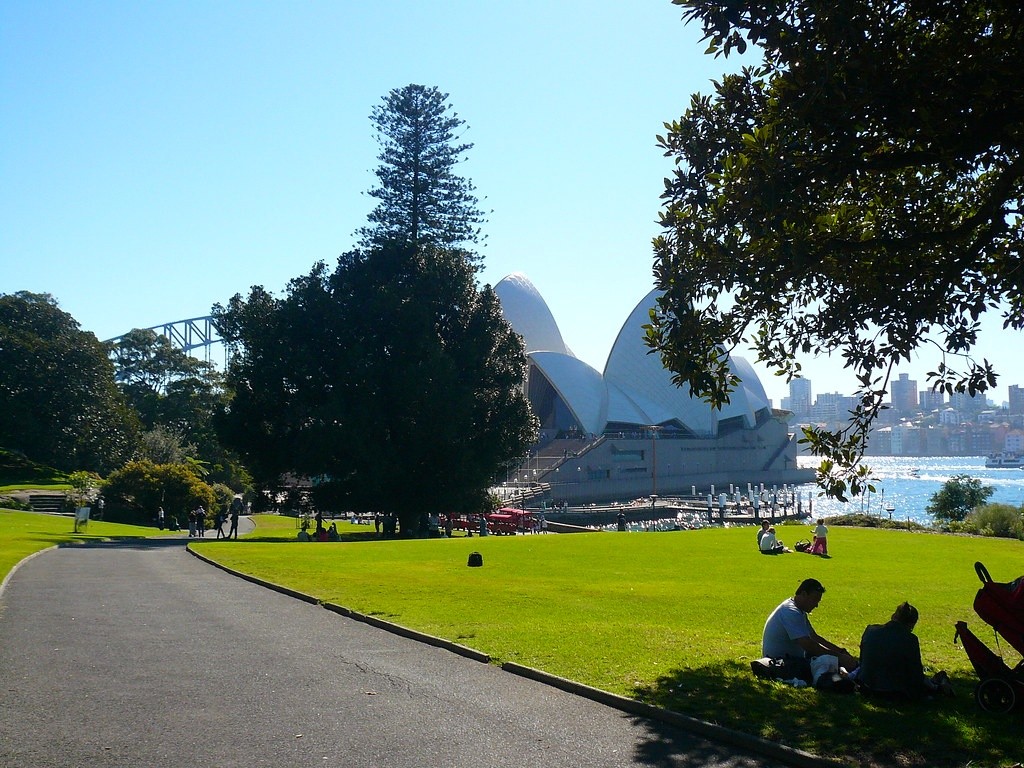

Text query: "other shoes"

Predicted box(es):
[823, 555, 830, 558]
[933, 671, 946, 684]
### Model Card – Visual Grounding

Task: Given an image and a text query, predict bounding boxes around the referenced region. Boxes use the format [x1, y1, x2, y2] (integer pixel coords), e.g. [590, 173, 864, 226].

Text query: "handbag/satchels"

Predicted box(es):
[800, 539, 811, 552]
[795, 542, 800, 551]
[751, 657, 774, 677]
[816, 673, 842, 685]
[769, 653, 813, 683]
[811, 654, 838, 684]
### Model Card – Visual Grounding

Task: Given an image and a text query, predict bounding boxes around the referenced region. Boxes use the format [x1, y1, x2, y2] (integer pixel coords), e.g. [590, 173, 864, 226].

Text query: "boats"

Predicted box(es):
[909, 468, 921, 472]
[985, 450, 1024, 468]
[910, 472, 919, 479]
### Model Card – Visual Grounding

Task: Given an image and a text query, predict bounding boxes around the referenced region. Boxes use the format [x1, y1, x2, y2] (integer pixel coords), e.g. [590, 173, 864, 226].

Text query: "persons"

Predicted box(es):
[542, 497, 563, 509]
[226, 509, 238, 539]
[478, 513, 488, 537]
[317, 511, 327, 528]
[358, 517, 362, 524]
[757, 520, 788, 555]
[810, 518, 828, 558]
[442, 515, 453, 538]
[617, 509, 626, 531]
[298, 526, 339, 542]
[857, 600, 949, 701]
[375, 514, 381, 538]
[158, 506, 164, 530]
[93, 496, 105, 520]
[420, 512, 441, 538]
[763, 579, 860, 680]
[351, 515, 355, 523]
[217, 510, 225, 539]
[189, 505, 206, 537]
[533, 515, 547, 535]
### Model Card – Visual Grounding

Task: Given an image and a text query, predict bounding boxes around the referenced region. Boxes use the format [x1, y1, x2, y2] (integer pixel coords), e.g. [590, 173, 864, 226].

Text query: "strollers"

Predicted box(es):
[955, 560, 1023, 717]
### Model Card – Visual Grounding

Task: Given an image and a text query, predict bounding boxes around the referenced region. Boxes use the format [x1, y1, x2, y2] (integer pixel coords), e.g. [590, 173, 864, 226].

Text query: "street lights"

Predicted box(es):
[648, 426, 661, 495]
[521, 490, 526, 535]
[650, 495, 658, 531]
